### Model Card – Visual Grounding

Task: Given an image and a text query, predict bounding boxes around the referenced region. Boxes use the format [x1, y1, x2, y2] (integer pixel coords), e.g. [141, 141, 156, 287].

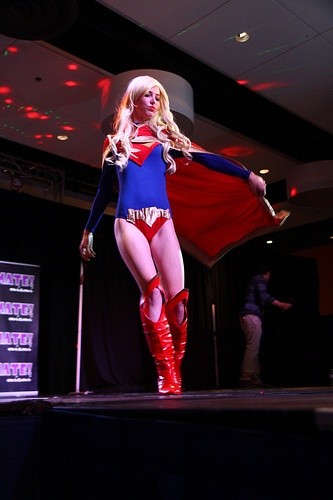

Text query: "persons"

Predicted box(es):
[235, 265, 293, 392]
[76, 74, 267, 399]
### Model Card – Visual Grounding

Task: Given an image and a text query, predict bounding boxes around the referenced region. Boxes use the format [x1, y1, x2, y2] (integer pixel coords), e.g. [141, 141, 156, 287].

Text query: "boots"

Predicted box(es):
[166, 289, 189, 367]
[139, 273, 183, 395]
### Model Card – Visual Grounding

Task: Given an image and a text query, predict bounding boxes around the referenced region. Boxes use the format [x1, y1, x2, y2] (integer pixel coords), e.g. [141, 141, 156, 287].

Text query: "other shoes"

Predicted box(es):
[239, 374, 265, 389]
